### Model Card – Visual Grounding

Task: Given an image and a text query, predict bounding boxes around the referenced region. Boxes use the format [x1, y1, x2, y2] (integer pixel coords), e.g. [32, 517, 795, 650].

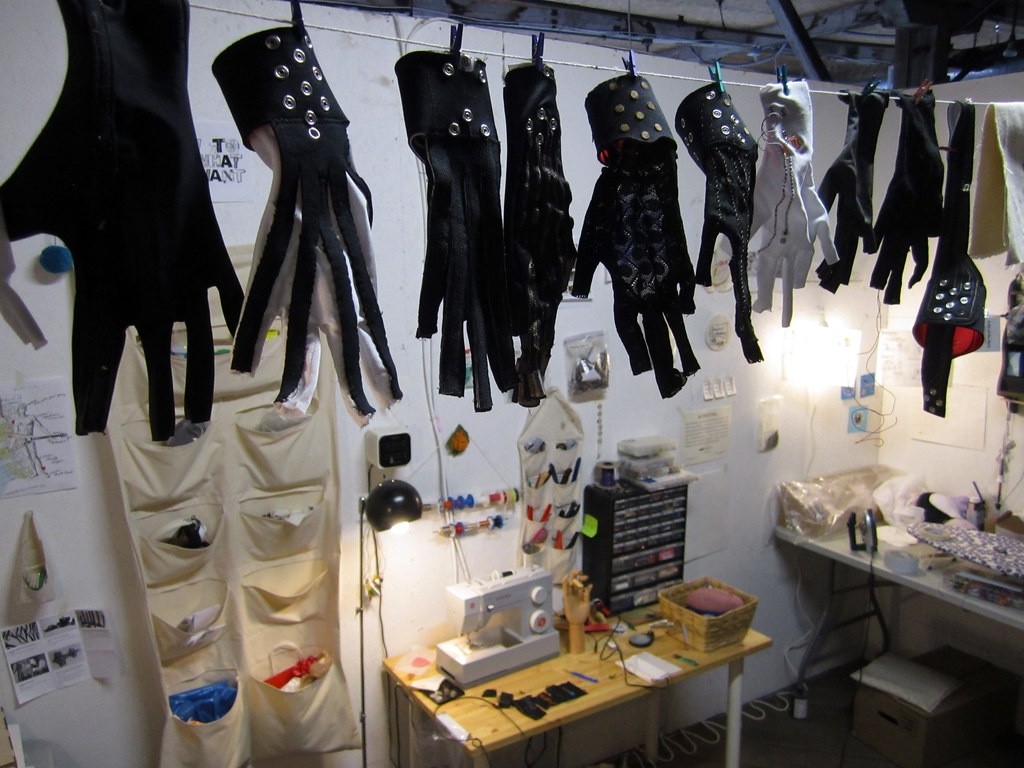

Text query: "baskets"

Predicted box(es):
[657, 576, 758, 652]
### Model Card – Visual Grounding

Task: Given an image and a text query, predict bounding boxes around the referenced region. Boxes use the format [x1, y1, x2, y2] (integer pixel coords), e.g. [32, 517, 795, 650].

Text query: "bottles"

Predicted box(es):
[790, 679, 808, 720]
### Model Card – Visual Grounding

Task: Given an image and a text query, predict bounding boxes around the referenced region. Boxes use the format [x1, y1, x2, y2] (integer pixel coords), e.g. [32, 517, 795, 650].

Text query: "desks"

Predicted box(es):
[381, 602, 772, 768]
[774, 517, 1024, 733]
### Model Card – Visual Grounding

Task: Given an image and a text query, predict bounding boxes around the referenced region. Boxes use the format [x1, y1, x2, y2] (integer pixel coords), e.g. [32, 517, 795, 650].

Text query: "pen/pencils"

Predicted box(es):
[672, 653, 698, 666]
[563, 669, 598, 682]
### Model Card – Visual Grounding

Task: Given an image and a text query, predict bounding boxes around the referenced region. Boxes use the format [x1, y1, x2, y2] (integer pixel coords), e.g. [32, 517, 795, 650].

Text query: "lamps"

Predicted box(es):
[360, 479, 424, 533]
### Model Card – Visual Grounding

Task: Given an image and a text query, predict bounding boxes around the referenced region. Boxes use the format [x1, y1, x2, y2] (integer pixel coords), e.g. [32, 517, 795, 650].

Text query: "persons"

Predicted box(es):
[562, 571, 593, 655]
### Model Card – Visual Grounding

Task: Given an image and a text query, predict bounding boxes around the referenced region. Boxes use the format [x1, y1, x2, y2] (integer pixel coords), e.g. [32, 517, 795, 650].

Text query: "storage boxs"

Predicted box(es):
[851, 643, 1023, 768]
[657, 577, 758, 653]
[582, 436, 701, 617]
